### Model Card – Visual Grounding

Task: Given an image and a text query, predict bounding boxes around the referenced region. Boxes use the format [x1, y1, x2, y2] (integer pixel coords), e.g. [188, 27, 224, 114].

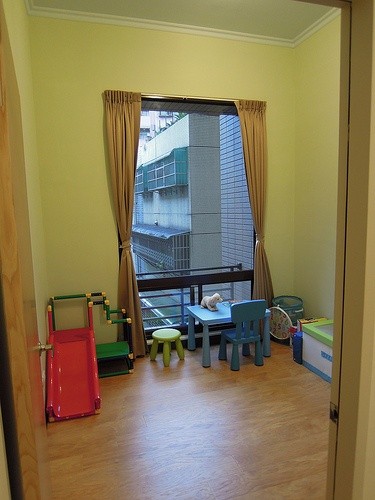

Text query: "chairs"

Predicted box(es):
[218, 300, 264, 371]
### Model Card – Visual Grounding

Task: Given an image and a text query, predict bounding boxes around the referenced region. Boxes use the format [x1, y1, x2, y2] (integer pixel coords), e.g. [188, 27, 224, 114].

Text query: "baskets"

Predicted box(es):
[273, 296, 304, 326]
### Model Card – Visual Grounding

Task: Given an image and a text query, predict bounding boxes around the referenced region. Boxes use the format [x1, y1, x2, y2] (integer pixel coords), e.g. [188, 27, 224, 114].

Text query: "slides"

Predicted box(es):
[47, 328, 101, 422]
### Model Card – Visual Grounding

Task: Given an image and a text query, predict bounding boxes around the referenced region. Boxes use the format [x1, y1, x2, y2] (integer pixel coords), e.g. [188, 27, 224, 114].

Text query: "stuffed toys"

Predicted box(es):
[200, 293, 223, 312]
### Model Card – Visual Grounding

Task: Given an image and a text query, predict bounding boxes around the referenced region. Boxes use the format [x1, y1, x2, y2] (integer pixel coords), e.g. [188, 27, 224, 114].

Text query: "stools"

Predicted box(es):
[150, 328, 185, 368]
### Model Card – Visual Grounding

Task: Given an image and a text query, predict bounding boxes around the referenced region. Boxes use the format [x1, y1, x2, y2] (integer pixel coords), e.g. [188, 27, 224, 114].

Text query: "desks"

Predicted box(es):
[185, 299, 271, 368]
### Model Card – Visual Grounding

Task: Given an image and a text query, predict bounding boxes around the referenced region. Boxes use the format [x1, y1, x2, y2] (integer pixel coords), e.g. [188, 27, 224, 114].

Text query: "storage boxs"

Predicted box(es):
[301, 319, 333, 384]
